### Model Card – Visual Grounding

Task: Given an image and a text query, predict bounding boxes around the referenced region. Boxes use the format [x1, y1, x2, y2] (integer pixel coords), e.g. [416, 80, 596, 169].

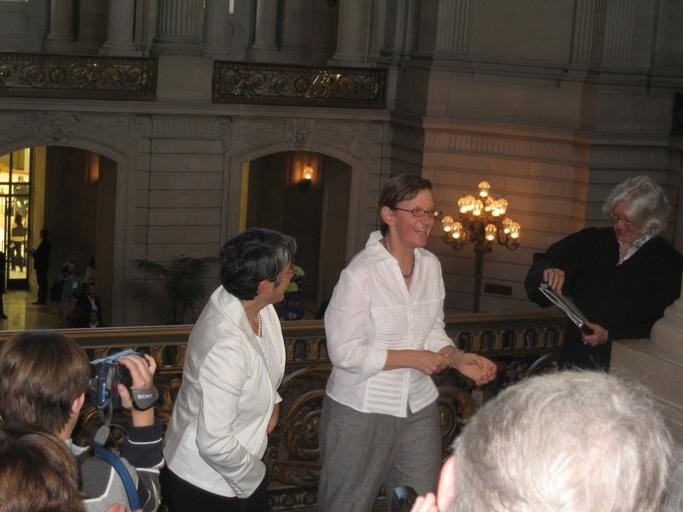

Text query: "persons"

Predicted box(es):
[406, 369, 682, 511]
[51, 258, 104, 327]
[283, 254, 305, 321]
[0, 429, 144, 512]
[0, 329, 164, 512]
[521, 175, 683, 373]
[154, 225, 299, 510]
[29, 228, 51, 305]
[0, 251, 9, 320]
[312, 172, 501, 511]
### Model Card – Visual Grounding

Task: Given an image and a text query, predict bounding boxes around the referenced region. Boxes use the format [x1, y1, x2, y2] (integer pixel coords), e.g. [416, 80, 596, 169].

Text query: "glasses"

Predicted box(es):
[271, 265, 298, 279]
[606, 211, 644, 227]
[392, 207, 443, 218]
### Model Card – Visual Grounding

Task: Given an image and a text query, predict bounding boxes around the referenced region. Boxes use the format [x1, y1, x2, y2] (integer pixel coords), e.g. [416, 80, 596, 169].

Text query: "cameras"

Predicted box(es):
[89, 349, 145, 413]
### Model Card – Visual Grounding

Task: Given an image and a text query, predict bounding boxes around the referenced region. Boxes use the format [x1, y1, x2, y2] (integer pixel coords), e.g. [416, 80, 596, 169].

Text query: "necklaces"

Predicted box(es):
[381, 239, 414, 279]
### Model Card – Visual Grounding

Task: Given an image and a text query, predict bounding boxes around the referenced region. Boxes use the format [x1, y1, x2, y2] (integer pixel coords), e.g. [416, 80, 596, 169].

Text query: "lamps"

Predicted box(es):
[304, 164, 315, 182]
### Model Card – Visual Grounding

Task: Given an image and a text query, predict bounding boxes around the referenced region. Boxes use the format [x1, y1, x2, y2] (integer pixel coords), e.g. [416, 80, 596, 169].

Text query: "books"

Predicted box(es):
[537, 282, 588, 329]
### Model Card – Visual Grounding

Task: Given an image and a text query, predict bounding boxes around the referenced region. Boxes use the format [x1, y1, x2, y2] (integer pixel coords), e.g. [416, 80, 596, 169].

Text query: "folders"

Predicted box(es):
[537, 282, 594, 335]
